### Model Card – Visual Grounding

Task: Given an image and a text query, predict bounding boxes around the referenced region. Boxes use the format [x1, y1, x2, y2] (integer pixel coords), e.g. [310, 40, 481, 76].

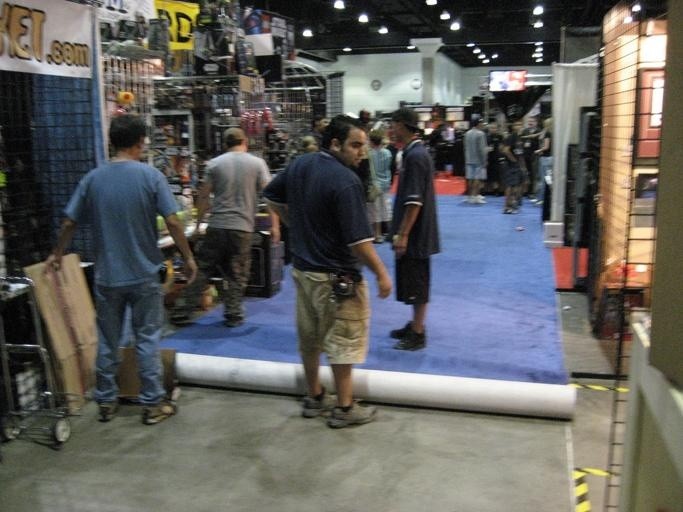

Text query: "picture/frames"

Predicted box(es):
[631, 68, 666, 164]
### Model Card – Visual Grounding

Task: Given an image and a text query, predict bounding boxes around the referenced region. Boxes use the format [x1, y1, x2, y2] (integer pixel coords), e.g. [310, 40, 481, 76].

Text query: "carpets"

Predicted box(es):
[159, 192, 578, 424]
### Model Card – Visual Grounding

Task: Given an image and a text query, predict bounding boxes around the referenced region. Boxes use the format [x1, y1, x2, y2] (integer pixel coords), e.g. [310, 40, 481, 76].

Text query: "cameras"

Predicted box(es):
[332, 276, 356, 298]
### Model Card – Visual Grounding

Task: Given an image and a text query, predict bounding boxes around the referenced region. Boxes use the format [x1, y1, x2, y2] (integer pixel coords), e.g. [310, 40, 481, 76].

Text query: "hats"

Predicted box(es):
[392, 107, 424, 133]
[223, 128, 246, 141]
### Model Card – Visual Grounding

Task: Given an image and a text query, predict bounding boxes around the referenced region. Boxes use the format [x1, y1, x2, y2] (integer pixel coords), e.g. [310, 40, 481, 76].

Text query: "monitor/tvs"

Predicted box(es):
[487, 69, 528, 91]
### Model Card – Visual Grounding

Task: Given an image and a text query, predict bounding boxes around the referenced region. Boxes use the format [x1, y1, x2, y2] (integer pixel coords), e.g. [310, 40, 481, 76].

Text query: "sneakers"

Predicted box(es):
[98, 404, 120, 421]
[393, 332, 425, 350]
[304, 389, 338, 417]
[327, 404, 377, 427]
[468, 194, 487, 204]
[170, 309, 193, 323]
[144, 400, 176, 423]
[225, 317, 244, 326]
[390, 321, 412, 338]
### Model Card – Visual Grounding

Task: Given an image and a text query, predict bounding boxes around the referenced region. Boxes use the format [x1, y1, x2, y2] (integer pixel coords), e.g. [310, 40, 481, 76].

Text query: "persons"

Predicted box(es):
[41, 113, 198, 425]
[168, 126, 281, 326]
[263, 111, 392, 431]
[383, 107, 441, 353]
[301, 101, 551, 245]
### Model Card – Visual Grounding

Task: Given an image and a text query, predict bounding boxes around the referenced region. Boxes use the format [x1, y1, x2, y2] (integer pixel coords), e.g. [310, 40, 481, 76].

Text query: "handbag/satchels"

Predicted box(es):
[367, 181, 384, 202]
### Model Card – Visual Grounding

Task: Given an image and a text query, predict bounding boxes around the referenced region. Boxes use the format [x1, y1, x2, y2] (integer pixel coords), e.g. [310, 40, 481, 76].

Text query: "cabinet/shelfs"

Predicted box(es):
[101, 51, 327, 212]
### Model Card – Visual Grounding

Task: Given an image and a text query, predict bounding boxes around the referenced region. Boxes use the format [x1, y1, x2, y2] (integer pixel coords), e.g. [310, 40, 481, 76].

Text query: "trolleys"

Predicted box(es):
[0, 273, 83, 445]
